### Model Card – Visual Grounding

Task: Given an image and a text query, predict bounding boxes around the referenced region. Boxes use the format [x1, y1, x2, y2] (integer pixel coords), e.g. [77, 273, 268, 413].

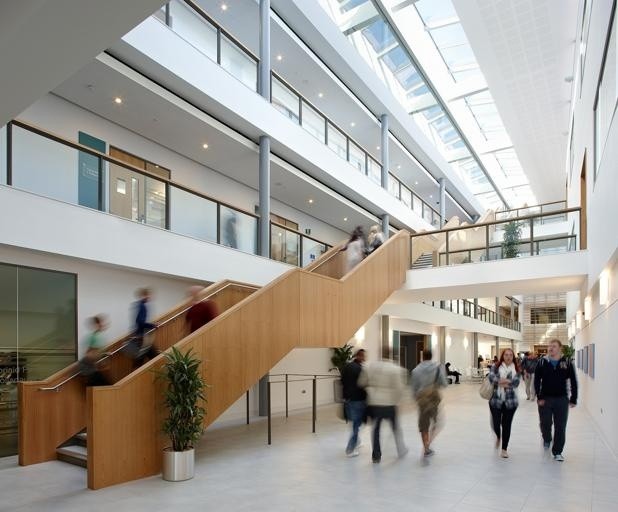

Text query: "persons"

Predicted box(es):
[443, 361, 462, 384]
[180, 284, 220, 334]
[339, 348, 369, 457]
[411, 348, 450, 456]
[357, 345, 409, 464]
[477, 349, 548, 402]
[74, 353, 120, 396]
[487, 348, 521, 460]
[126, 285, 159, 366]
[532, 339, 579, 462]
[86, 314, 114, 371]
[337, 221, 386, 271]
[223, 211, 239, 249]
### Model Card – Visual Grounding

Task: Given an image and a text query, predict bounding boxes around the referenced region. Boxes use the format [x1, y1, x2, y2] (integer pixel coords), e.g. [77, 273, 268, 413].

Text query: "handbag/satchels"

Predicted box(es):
[479, 377, 496, 401]
[415, 383, 441, 412]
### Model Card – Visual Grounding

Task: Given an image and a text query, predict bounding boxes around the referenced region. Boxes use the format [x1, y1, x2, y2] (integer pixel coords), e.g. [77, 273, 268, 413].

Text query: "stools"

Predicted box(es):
[443, 375, 456, 385]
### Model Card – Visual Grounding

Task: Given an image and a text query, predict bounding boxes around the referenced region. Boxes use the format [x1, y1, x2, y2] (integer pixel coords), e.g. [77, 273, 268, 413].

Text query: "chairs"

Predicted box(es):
[469, 367, 481, 384]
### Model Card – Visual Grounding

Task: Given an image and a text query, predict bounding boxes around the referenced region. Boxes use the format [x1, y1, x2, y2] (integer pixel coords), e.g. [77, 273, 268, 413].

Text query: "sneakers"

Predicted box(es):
[543, 440, 551, 451]
[554, 453, 564, 462]
[501, 451, 509, 459]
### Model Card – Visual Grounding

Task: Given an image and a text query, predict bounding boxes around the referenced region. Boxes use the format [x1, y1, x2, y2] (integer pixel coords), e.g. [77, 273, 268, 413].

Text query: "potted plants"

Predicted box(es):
[148, 341, 213, 483]
[329, 341, 354, 403]
